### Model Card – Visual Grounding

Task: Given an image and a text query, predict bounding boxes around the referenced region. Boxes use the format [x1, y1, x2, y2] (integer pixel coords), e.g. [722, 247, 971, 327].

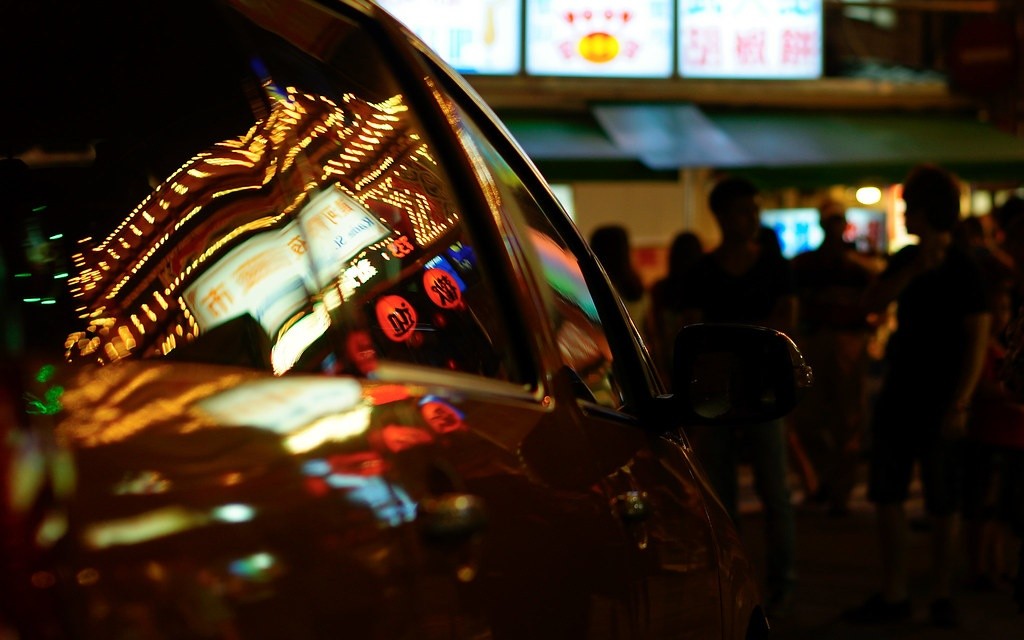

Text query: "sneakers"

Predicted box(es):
[900, 584, 960, 626]
[839, 589, 913, 624]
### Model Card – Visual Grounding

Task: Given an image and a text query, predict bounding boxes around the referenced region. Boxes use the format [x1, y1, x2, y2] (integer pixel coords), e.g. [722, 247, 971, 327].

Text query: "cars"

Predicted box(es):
[0, 0, 811, 640]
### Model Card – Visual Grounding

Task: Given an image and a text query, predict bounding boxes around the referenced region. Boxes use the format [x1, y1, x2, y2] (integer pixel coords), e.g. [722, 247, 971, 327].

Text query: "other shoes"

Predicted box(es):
[826, 500, 851, 518]
[805, 478, 839, 503]
[762, 580, 794, 621]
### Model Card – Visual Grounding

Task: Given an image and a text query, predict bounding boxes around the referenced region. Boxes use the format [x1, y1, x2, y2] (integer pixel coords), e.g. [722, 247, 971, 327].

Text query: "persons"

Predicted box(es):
[588, 162, 1024, 629]
